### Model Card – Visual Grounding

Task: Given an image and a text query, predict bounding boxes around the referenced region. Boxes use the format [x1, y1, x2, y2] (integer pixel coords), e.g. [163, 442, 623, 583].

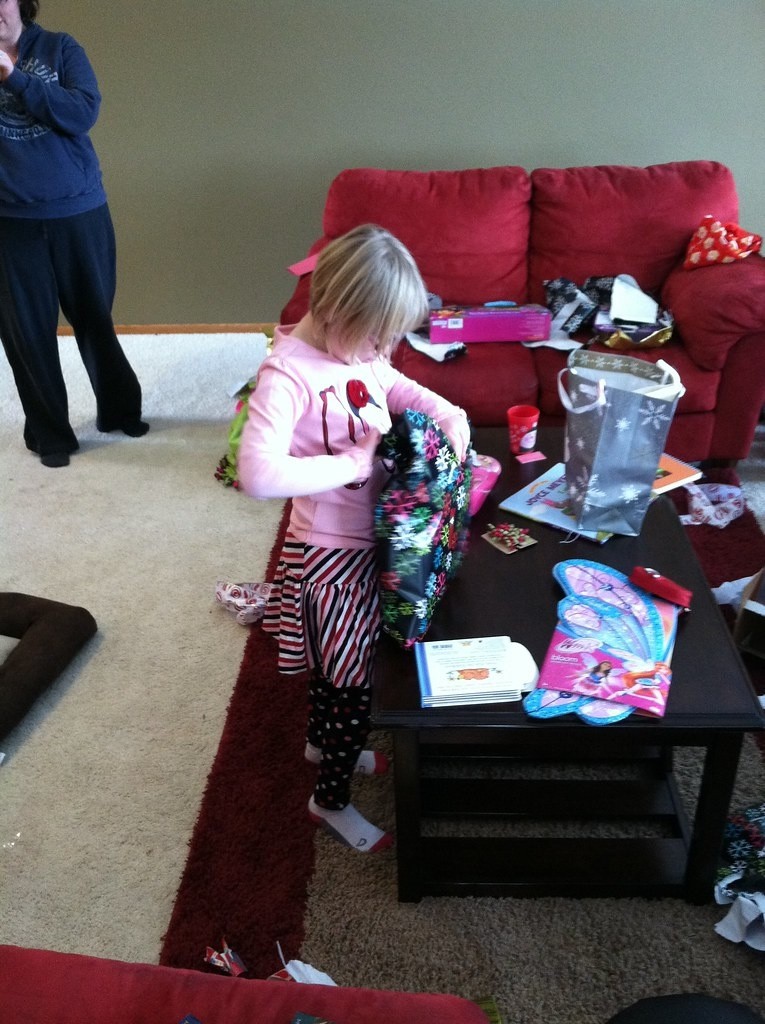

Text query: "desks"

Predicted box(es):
[364, 425, 765, 904]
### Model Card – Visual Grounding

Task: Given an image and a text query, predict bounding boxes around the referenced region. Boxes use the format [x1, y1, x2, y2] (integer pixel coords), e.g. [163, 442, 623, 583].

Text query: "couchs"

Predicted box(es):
[277, 158, 764, 487]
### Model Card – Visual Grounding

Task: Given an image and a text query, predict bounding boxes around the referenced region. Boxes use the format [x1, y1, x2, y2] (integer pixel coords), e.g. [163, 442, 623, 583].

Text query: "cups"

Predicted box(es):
[508, 405, 540, 455]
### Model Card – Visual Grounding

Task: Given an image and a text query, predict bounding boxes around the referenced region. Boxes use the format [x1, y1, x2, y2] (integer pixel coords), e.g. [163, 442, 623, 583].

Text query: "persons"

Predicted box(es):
[1, 0, 151, 468]
[235, 222, 471, 855]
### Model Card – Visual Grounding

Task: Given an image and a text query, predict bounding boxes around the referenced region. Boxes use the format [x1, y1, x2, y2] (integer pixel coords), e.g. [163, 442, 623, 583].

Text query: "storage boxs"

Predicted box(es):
[557, 346, 685, 539]
[427, 302, 552, 345]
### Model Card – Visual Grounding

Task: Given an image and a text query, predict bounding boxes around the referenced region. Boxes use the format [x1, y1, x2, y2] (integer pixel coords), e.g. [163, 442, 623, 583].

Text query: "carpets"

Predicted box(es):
[0, 332, 765, 1024]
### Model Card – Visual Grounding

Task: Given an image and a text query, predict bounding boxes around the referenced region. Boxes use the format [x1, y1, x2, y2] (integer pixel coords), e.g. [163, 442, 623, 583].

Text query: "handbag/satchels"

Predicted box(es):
[556, 349, 687, 537]
